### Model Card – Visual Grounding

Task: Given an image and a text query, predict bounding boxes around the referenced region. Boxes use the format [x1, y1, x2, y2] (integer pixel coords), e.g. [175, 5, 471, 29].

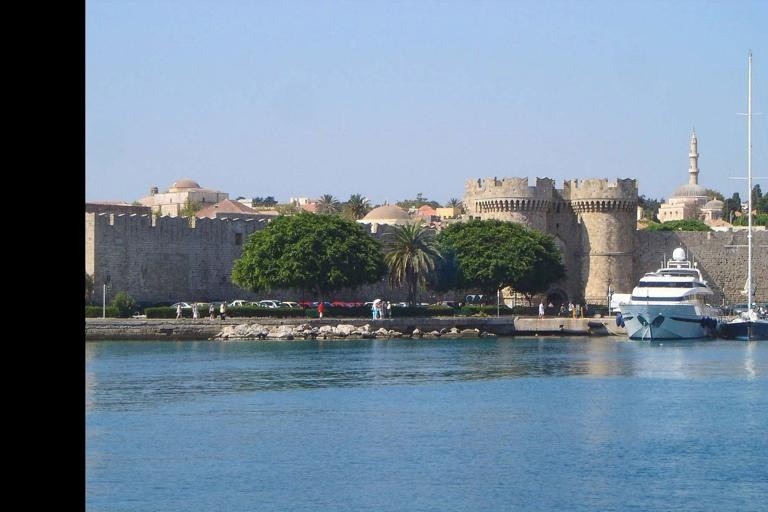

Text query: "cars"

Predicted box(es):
[713, 302, 767, 319]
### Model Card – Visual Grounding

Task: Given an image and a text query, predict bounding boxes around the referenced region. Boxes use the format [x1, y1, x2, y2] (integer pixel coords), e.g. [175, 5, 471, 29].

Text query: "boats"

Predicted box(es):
[619, 246, 723, 342]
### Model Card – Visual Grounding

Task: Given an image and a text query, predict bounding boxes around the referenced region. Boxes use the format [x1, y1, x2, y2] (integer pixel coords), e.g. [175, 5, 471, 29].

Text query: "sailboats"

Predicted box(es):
[717, 50, 768, 342]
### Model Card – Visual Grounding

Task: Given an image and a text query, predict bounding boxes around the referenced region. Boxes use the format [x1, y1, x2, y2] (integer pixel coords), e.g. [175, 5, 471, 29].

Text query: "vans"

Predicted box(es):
[610, 293, 630, 316]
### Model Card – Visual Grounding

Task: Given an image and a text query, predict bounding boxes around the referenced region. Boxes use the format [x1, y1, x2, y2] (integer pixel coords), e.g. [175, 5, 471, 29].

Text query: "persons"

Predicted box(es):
[318, 301, 324, 318]
[176, 302, 227, 318]
[372, 300, 392, 318]
[538, 301, 589, 318]
